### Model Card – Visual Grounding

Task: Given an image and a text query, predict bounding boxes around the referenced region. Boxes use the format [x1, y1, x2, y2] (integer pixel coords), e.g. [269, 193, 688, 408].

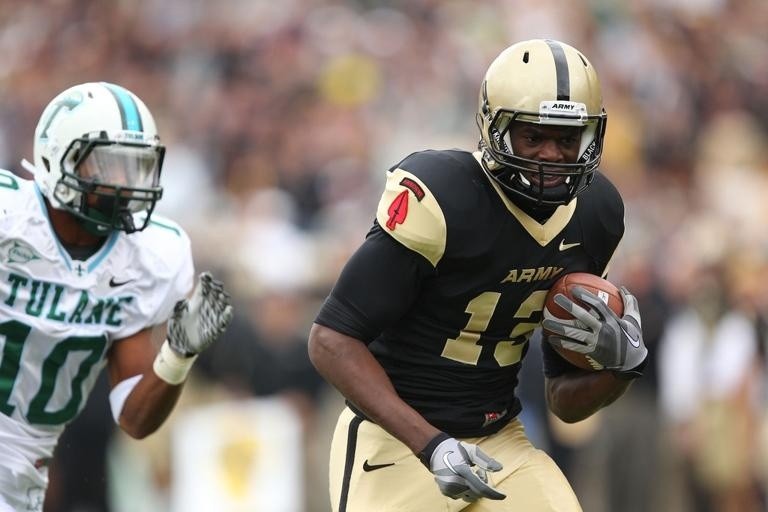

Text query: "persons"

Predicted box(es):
[0, 80, 235, 512]
[307, 38, 650, 512]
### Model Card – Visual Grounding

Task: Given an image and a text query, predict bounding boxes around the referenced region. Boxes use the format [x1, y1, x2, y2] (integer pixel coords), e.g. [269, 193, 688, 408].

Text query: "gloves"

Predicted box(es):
[166, 272, 233, 358]
[417, 431, 507, 502]
[543, 286, 648, 379]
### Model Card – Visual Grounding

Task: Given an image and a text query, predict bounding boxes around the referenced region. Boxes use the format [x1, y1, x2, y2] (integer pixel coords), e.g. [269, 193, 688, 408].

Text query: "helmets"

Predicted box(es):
[475, 39, 606, 205]
[34, 81, 166, 234]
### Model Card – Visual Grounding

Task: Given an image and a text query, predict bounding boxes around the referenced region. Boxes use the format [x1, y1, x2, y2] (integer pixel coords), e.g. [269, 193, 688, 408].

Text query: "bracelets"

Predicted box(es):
[153, 338, 200, 385]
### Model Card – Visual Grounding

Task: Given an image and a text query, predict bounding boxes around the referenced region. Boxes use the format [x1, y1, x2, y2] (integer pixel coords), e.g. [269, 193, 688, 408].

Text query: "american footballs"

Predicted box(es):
[543, 272, 624, 374]
